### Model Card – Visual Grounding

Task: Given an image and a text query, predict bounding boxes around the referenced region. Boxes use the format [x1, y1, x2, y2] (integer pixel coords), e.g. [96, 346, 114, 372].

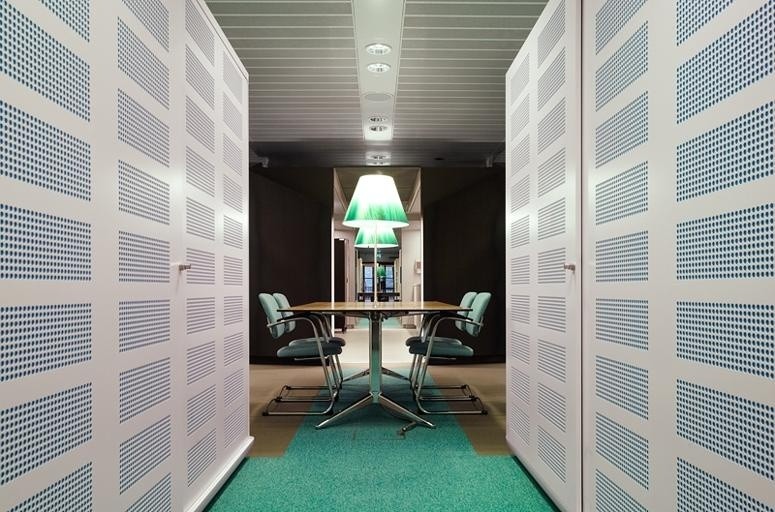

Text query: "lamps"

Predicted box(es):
[355, 227, 398, 304]
[341, 176, 409, 307]
[377, 267, 387, 291]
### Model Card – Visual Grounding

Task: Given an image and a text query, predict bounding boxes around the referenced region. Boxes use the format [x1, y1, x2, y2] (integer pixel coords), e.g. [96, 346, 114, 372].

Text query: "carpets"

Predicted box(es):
[355, 318, 402, 329]
[334, 329, 424, 364]
[204, 369, 554, 512]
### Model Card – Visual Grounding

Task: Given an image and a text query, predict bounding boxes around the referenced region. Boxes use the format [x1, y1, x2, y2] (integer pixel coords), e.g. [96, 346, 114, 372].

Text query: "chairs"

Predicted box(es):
[410, 291, 477, 399]
[259, 294, 338, 415]
[414, 292, 491, 414]
[274, 292, 343, 399]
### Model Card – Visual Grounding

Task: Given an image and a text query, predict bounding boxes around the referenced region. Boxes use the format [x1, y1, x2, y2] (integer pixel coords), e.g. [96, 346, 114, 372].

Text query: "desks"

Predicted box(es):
[278, 302, 472, 430]
